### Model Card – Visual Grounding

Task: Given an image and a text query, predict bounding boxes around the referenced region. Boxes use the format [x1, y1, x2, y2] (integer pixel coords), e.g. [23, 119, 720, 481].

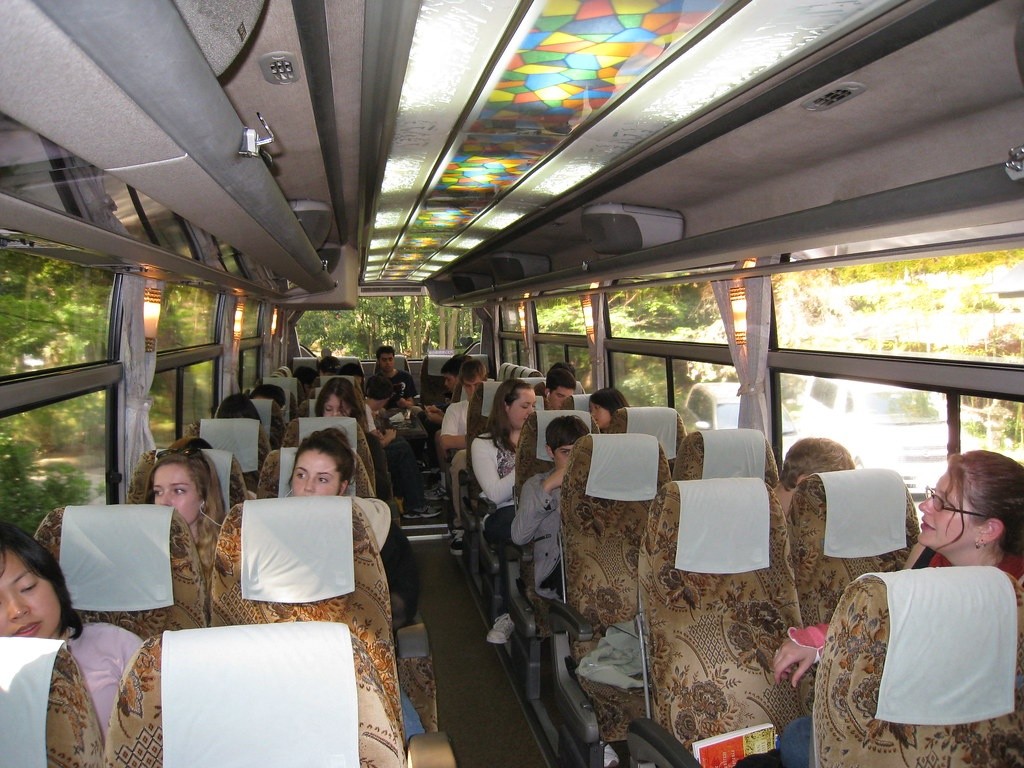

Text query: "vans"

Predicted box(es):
[798, 373, 952, 495]
[685, 381, 800, 464]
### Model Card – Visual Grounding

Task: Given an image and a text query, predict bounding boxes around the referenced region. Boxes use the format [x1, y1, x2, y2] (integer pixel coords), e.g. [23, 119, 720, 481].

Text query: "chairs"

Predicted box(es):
[0, 355, 1024, 768]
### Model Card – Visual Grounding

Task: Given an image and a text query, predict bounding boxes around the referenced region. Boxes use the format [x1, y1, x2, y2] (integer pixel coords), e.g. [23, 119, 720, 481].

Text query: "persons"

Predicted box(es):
[471, 362, 628, 649]
[772, 448, 1024, 768]
[286, 425, 426, 747]
[169, 437, 213, 448]
[0, 521, 144, 751]
[424, 350, 487, 557]
[774, 435, 855, 519]
[141, 449, 227, 620]
[211, 344, 449, 521]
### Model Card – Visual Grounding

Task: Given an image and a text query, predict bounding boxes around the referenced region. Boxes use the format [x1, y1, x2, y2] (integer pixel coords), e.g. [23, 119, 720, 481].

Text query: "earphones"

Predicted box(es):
[200, 501, 205, 507]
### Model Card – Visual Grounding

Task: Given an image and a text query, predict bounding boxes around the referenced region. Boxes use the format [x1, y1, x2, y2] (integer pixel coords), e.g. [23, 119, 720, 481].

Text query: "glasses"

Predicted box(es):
[925, 485, 989, 518]
[157, 448, 204, 461]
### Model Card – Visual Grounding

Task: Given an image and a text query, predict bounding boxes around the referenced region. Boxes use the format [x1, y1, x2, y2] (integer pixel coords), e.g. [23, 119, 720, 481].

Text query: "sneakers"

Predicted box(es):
[407, 502, 442, 519]
[486, 612, 515, 643]
[425, 485, 446, 501]
[449, 528, 465, 556]
